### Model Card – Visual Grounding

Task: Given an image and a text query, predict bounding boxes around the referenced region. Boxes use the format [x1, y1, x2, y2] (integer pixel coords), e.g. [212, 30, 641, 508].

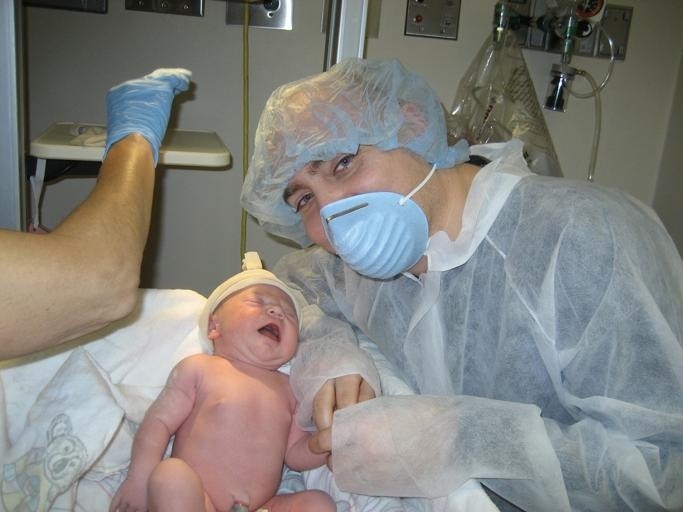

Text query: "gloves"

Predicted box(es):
[101, 67, 193, 169]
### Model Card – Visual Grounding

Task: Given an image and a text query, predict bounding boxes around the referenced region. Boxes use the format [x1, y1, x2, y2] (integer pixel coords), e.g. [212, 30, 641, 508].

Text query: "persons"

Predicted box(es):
[237, 55, 682, 512]
[107, 267, 345, 511]
[1, 66, 194, 365]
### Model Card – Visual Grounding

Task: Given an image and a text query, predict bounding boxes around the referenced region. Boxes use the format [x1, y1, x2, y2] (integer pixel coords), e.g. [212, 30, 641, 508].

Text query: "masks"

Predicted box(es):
[319, 162, 437, 281]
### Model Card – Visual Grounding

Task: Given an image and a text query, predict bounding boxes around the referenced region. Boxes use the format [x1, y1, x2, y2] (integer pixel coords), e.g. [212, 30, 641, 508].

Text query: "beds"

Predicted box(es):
[0, 289, 501, 512]
